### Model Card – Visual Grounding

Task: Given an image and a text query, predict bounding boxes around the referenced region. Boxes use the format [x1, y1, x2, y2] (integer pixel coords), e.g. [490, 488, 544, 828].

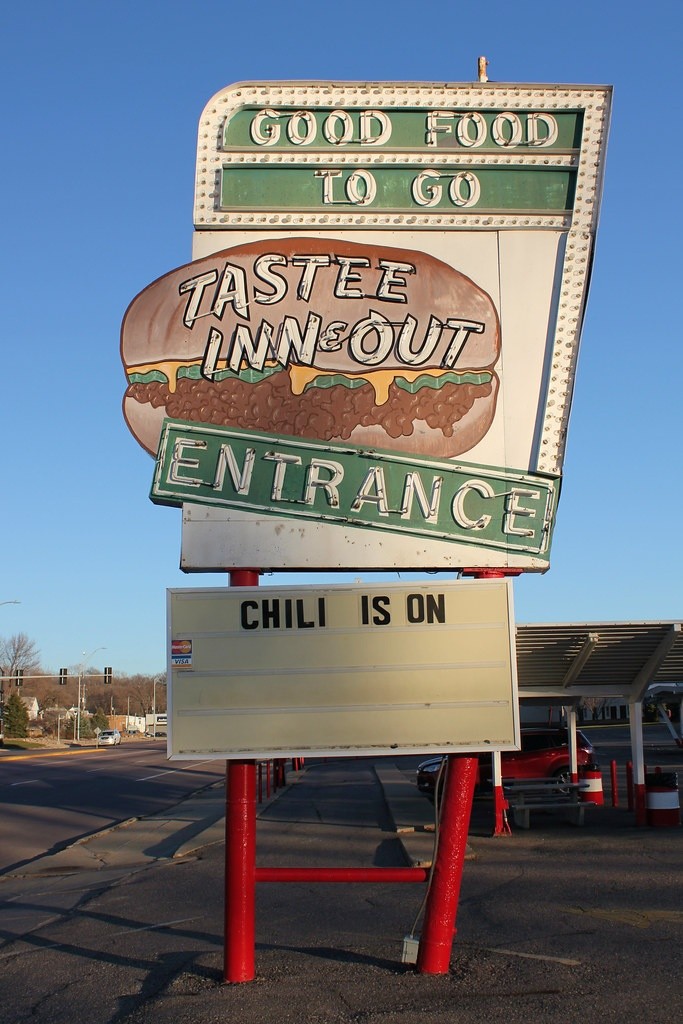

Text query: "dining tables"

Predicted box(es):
[488, 775, 564, 804]
[503, 782, 591, 819]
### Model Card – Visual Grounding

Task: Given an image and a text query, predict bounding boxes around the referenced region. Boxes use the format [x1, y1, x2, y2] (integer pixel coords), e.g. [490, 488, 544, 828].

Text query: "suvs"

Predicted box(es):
[417, 728, 600, 805]
[98, 729, 122, 746]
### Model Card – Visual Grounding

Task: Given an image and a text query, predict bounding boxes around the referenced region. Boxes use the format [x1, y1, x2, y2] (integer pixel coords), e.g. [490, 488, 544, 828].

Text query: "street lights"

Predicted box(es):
[76, 647, 107, 741]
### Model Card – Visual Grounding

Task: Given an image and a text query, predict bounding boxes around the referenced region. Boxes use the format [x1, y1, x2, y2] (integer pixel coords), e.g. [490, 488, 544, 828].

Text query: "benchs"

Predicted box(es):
[511, 800, 596, 830]
